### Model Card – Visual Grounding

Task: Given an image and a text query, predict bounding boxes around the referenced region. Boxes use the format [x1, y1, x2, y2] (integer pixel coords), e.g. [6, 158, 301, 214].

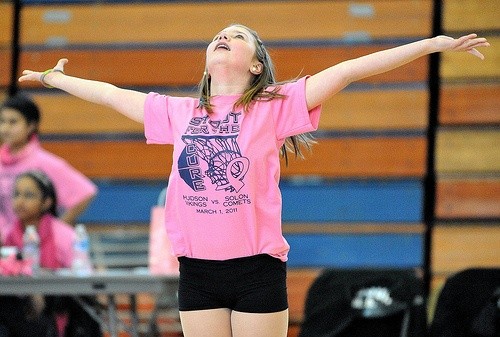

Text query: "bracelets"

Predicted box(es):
[40, 69, 65, 89]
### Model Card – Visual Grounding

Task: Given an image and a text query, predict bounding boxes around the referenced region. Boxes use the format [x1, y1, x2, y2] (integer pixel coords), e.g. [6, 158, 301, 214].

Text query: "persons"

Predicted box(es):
[0, 93, 99, 243]
[0, 169, 94, 277]
[17, 24, 490, 337]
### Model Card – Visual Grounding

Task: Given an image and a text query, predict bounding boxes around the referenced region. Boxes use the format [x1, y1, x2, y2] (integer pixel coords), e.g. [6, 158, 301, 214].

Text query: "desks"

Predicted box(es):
[0, 269, 179, 337]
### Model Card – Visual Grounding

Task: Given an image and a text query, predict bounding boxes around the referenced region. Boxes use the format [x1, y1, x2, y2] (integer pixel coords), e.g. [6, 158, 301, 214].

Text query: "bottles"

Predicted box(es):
[71, 224, 91, 277]
[22, 225, 40, 277]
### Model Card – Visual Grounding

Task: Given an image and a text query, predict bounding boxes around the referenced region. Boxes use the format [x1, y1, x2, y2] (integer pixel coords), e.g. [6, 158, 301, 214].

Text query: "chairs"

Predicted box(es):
[430, 268, 500, 337]
[298, 269, 428, 337]
